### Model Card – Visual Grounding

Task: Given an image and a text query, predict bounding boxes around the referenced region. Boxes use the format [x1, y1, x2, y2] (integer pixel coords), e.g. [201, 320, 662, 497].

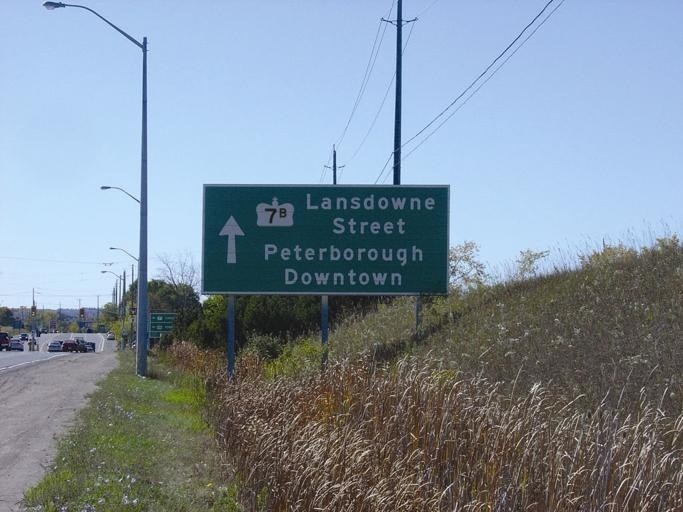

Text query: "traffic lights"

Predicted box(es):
[79, 308, 83, 319]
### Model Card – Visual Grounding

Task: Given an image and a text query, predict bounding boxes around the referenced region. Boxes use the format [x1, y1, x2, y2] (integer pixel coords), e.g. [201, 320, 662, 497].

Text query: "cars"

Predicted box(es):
[6, 338, 24, 351]
[0, 332, 11, 352]
[17, 333, 28, 341]
[38, 327, 47, 334]
[46, 336, 95, 353]
[106, 331, 115, 340]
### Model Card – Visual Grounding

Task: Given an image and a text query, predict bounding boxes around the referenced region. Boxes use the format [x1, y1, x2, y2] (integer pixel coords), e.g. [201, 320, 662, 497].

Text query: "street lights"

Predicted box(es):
[43, 1, 149, 378]
[108, 247, 141, 353]
[99, 185, 144, 359]
[100, 270, 126, 351]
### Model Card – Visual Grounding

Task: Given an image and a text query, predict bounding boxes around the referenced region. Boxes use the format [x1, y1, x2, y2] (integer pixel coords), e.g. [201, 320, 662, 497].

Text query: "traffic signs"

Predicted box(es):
[201, 185, 448, 296]
[149, 313, 177, 323]
[149, 324, 179, 332]
[31, 306, 35, 317]
